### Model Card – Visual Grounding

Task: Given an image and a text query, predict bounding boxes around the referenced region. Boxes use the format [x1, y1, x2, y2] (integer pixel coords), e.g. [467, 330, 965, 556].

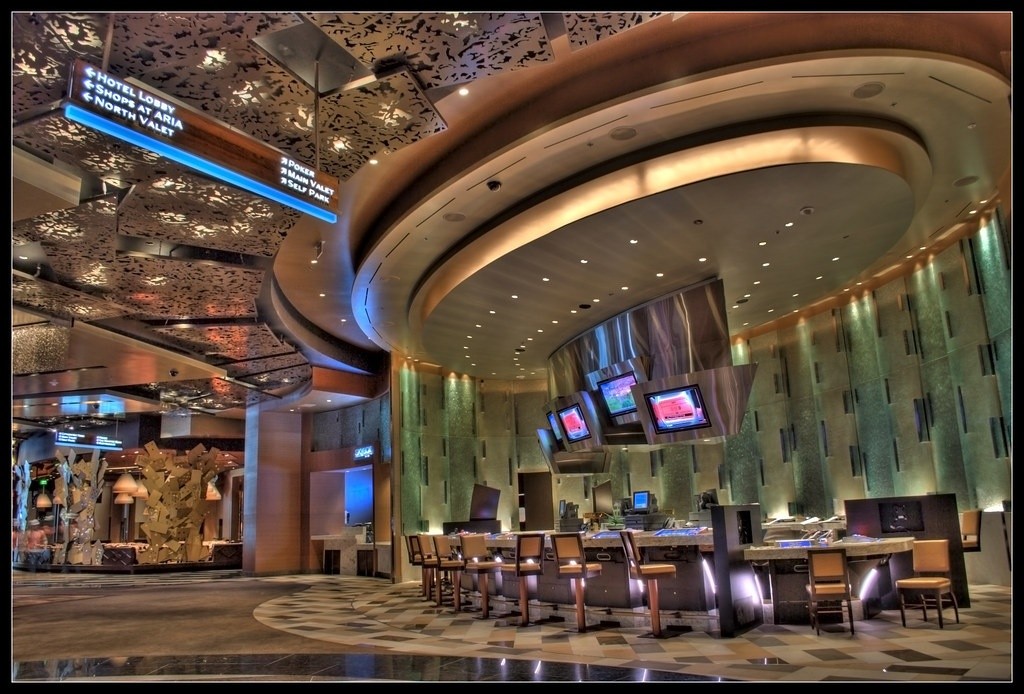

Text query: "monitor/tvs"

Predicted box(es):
[559, 499, 566, 516]
[535, 371, 713, 475]
[706, 488, 719, 505]
[632, 491, 650, 512]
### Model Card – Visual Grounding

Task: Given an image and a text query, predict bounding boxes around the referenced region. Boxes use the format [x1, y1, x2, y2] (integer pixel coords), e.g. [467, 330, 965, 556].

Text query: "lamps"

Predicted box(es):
[205, 481, 222, 501]
[36, 479, 52, 508]
[53, 496, 62, 505]
[112, 469, 149, 505]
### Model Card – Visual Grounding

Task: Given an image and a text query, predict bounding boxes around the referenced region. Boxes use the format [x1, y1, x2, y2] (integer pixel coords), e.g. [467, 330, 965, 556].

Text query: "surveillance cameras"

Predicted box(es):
[169, 371, 179, 377]
[487, 181, 501, 192]
[92, 404, 99, 409]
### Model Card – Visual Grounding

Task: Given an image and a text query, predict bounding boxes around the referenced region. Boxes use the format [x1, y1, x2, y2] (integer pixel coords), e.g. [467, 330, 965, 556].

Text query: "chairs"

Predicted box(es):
[960, 511, 981, 552]
[805, 548, 855, 637]
[894, 539, 960, 629]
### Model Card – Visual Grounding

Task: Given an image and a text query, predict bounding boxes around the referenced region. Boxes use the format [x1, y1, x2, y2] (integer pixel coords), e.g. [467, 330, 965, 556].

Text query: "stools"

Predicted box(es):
[550, 533, 621, 634]
[619, 530, 693, 640]
[500, 534, 546, 628]
[460, 534, 501, 620]
[404, 535, 480, 615]
